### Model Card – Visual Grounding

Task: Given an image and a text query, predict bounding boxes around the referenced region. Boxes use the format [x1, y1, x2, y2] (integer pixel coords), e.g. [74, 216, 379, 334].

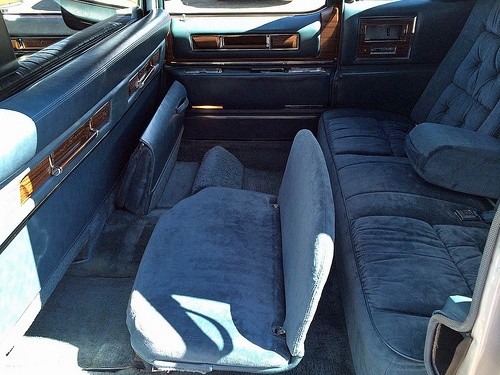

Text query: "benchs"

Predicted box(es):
[1, 7, 173, 361]
[318, 0, 500, 374]
[124, 128, 336, 373]
